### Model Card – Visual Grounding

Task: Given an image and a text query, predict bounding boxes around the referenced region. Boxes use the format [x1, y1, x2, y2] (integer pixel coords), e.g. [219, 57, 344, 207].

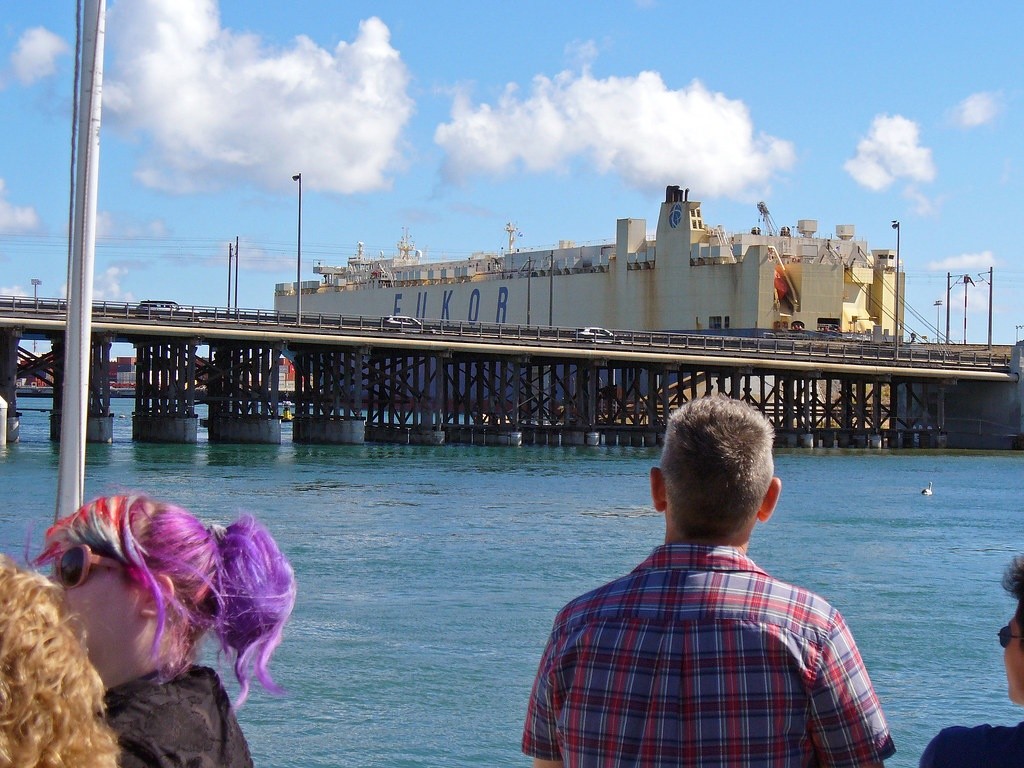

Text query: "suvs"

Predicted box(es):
[135, 300, 200, 322]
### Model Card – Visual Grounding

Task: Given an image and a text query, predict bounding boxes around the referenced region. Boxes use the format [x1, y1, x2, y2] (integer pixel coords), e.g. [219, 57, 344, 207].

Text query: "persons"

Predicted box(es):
[521, 394, 896, 768]
[920, 553, 1024, 768]
[0, 494, 296, 768]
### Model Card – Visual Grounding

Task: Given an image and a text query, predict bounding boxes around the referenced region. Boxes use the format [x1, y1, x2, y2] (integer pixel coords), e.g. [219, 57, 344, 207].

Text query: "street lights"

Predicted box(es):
[292, 173, 302, 328]
[891, 220, 899, 362]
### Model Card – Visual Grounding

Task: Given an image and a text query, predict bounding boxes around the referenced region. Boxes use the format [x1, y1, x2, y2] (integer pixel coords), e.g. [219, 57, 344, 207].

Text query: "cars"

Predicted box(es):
[380, 315, 436, 335]
[571, 327, 625, 345]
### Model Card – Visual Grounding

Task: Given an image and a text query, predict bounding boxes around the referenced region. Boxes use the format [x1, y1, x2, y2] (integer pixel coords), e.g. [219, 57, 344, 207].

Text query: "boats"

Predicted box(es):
[274, 185, 905, 429]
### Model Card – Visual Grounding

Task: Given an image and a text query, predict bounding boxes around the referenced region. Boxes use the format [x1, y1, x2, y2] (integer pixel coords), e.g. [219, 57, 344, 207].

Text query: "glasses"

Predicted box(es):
[57, 543, 122, 590]
[998, 625, 1023, 648]
